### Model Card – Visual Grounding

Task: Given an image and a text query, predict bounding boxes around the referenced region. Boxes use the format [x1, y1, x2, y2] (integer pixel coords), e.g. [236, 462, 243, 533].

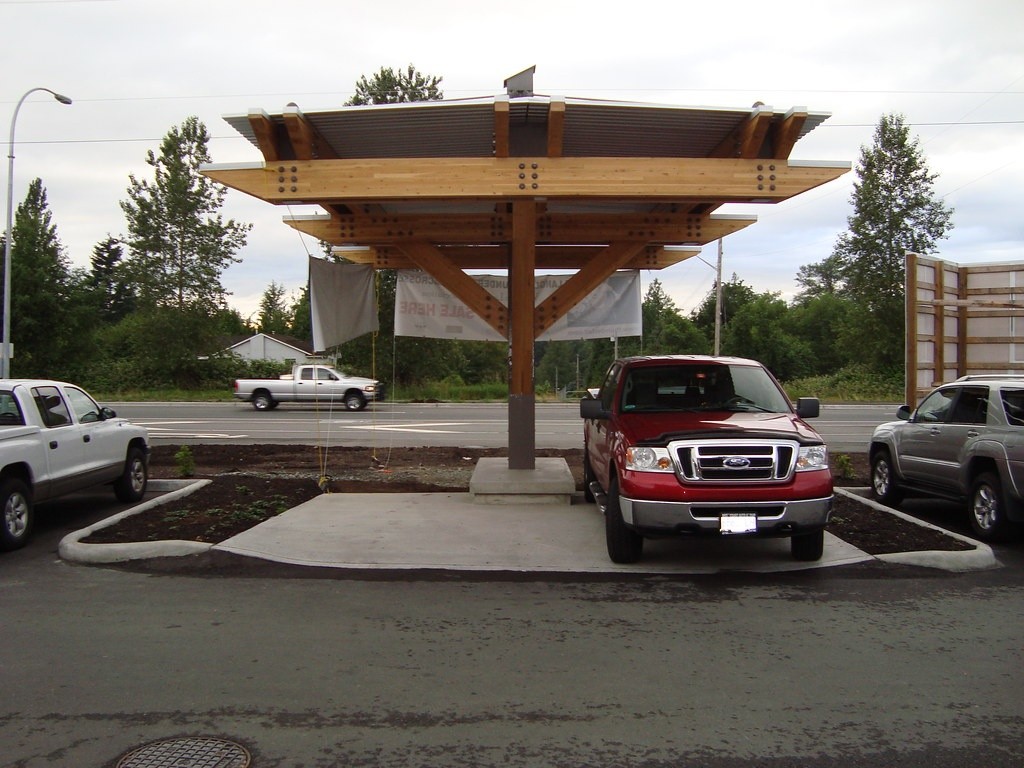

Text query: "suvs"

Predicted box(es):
[866, 373, 1024, 539]
[0, 378, 151, 554]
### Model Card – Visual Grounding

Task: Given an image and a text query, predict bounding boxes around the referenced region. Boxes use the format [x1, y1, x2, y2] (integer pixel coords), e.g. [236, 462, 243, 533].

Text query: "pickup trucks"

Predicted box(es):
[578, 354, 836, 565]
[233, 364, 385, 411]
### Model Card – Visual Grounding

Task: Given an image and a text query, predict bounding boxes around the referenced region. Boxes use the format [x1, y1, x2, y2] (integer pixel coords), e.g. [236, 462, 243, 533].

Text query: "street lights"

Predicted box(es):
[2, 85, 75, 380]
